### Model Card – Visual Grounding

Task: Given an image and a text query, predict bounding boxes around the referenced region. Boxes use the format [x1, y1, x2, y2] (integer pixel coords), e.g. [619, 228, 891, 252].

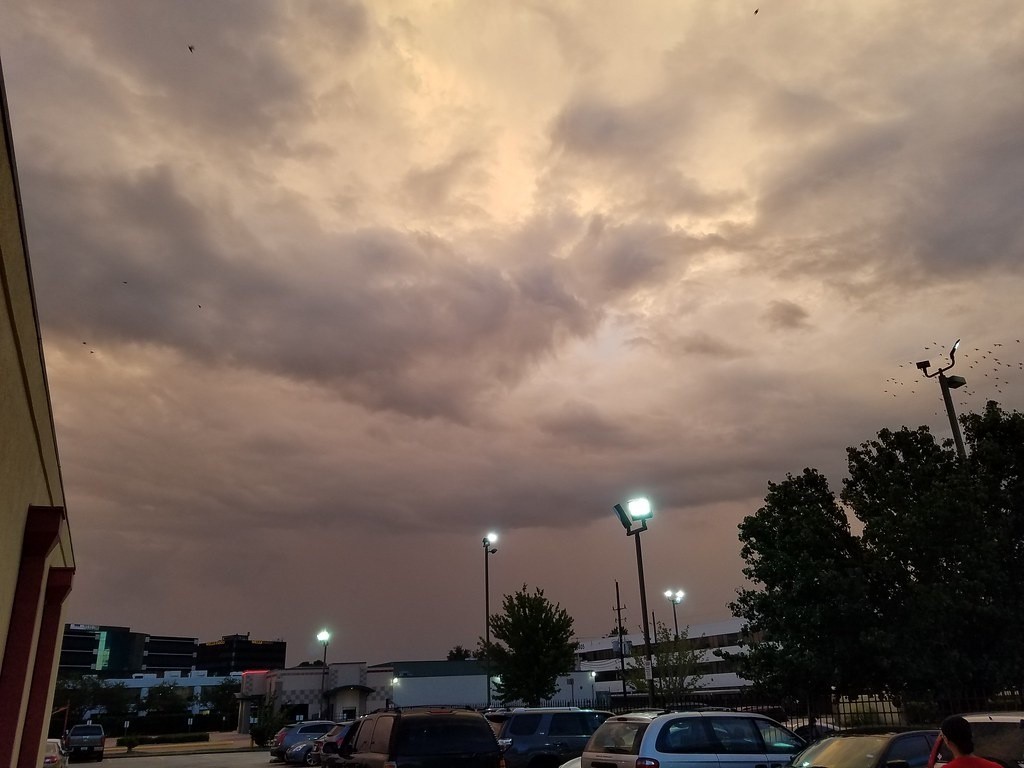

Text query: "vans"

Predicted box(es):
[582, 707, 808, 768]
[929, 712, 1024, 768]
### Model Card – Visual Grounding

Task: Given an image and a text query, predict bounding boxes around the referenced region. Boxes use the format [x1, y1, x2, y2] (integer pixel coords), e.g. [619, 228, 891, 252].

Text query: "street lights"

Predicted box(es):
[663, 590, 684, 639]
[317, 630, 329, 719]
[915, 340, 970, 461]
[483, 534, 497, 706]
[613, 496, 656, 708]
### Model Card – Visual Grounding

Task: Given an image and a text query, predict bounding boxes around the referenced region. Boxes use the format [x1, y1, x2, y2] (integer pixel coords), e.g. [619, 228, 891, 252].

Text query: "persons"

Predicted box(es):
[940, 716, 1004, 768]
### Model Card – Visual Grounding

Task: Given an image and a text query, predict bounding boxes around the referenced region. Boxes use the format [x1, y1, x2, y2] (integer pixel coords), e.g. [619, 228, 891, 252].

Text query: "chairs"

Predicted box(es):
[730, 726, 754, 753]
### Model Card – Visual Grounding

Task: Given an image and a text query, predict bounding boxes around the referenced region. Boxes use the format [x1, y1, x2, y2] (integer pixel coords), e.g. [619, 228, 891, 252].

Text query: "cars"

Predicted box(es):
[286, 738, 319, 768]
[44, 741, 66, 768]
[311, 721, 353, 763]
[785, 728, 942, 768]
[558, 725, 793, 768]
[270, 721, 337, 758]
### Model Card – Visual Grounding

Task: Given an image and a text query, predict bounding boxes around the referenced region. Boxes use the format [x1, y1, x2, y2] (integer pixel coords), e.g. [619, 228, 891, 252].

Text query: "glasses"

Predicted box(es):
[939, 733, 946, 739]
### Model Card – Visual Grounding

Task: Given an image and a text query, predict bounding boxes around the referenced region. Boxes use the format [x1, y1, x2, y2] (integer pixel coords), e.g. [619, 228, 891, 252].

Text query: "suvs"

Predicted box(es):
[317, 706, 505, 768]
[486, 706, 632, 768]
[66, 724, 105, 760]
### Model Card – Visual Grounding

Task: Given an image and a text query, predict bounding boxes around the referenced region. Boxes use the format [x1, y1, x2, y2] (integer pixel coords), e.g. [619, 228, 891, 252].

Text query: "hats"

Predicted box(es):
[941, 715, 974, 754]
